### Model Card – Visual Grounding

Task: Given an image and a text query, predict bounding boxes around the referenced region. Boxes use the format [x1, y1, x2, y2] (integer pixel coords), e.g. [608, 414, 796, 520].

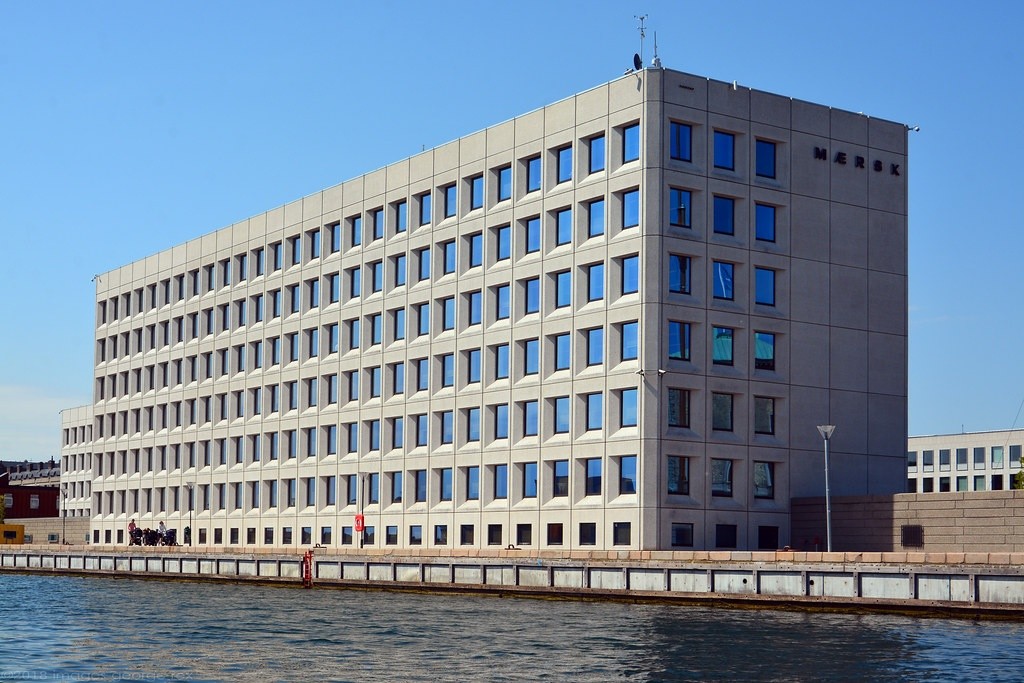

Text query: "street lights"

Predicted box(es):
[816, 424, 836, 552]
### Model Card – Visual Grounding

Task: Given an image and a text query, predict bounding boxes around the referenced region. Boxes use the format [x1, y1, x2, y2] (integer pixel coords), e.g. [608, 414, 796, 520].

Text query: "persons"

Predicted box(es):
[128, 519, 138, 544]
[157, 520, 166, 546]
